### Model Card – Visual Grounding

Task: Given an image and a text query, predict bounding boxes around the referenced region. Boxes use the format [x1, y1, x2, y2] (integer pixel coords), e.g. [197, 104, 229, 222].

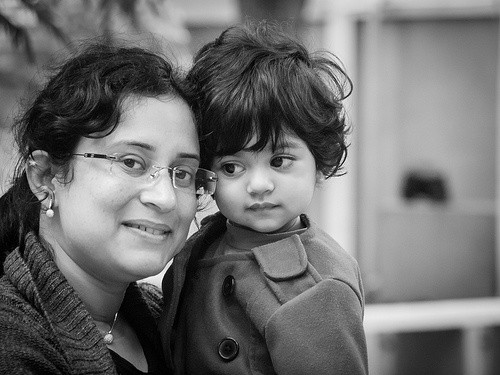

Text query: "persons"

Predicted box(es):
[150, 20, 369, 375]
[1, 31, 201, 375]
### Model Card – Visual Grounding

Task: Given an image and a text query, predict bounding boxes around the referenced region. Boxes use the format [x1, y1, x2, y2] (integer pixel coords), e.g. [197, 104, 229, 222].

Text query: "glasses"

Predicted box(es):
[62, 151, 218, 195]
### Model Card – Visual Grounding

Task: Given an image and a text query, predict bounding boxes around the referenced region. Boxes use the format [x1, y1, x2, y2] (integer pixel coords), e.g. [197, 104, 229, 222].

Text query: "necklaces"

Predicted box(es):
[103, 282, 130, 344]
